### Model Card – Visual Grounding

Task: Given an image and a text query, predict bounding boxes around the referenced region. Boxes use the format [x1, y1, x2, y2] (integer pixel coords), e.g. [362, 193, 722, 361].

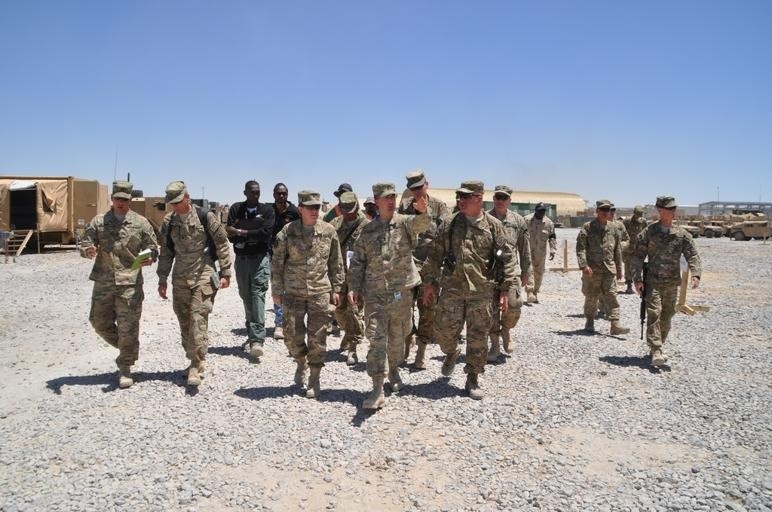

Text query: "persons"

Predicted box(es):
[224, 169, 559, 412]
[154, 178, 234, 387]
[75, 180, 161, 388]
[574, 197, 650, 337]
[631, 191, 703, 369]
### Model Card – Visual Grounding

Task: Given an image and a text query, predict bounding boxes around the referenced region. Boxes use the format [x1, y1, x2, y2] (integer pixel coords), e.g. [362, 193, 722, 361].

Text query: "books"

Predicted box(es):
[130, 247, 152, 272]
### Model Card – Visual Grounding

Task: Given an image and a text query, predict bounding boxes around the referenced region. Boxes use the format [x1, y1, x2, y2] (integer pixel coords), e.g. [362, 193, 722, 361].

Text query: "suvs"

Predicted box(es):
[641, 206, 772, 241]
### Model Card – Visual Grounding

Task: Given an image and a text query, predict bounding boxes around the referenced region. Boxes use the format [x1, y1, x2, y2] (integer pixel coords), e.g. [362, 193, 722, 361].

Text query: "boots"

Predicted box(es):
[626, 280, 634, 294]
[649, 346, 669, 366]
[119, 366, 133, 388]
[585, 316, 594, 332]
[243, 322, 513, 409]
[527, 291, 536, 303]
[181, 358, 206, 386]
[611, 319, 630, 335]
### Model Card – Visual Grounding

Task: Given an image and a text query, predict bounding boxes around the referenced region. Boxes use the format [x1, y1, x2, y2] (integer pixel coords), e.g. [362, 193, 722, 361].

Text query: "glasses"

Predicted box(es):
[276, 191, 288, 196]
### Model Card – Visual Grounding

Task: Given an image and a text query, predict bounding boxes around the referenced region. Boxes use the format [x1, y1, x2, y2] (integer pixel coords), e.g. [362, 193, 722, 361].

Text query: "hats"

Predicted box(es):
[166, 181, 187, 204]
[113, 180, 133, 200]
[597, 195, 678, 218]
[298, 170, 546, 214]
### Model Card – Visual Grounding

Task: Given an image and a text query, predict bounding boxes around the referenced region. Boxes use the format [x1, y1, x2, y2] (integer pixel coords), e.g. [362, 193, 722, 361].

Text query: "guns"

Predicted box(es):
[486, 236, 509, 283]
[640, 262, 649, 340]
